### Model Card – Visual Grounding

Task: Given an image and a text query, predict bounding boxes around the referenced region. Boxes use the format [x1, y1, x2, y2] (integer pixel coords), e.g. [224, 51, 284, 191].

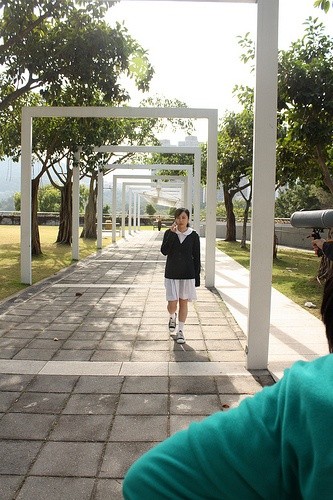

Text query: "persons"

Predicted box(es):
[156, 215, 162, 231]
[161, 208, 201, 343]
[122, 236, 333, 500]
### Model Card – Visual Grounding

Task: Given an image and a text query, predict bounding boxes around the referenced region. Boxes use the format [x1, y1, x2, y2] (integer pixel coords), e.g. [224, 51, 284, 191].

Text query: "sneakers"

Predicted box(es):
[168, 312, 177, 330]
[177, 330, 185, 343]
